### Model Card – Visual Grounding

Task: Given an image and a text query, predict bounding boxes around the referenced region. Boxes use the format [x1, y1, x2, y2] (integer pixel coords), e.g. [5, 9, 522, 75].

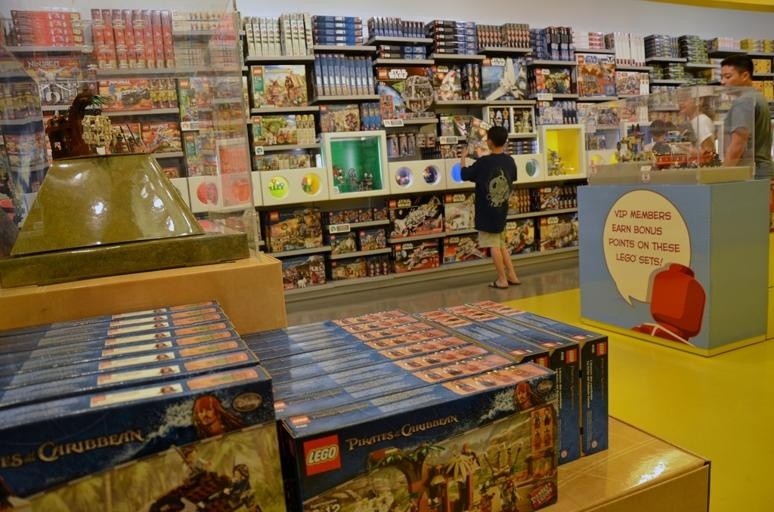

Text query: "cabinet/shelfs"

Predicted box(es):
[0, 34, 774, 298]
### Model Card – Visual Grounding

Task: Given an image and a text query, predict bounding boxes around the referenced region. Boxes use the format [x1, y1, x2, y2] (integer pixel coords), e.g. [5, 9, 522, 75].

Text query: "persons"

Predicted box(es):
[642, 118, 680, 156]
[459, 124, 523, 290]
[718, 55, 774, 179]
[676, 84, 717, 155]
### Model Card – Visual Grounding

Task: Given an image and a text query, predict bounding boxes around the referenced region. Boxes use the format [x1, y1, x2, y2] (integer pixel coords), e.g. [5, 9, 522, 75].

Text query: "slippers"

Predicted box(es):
[488, 280, 509, 289]
[506, 280, 522, 285]
